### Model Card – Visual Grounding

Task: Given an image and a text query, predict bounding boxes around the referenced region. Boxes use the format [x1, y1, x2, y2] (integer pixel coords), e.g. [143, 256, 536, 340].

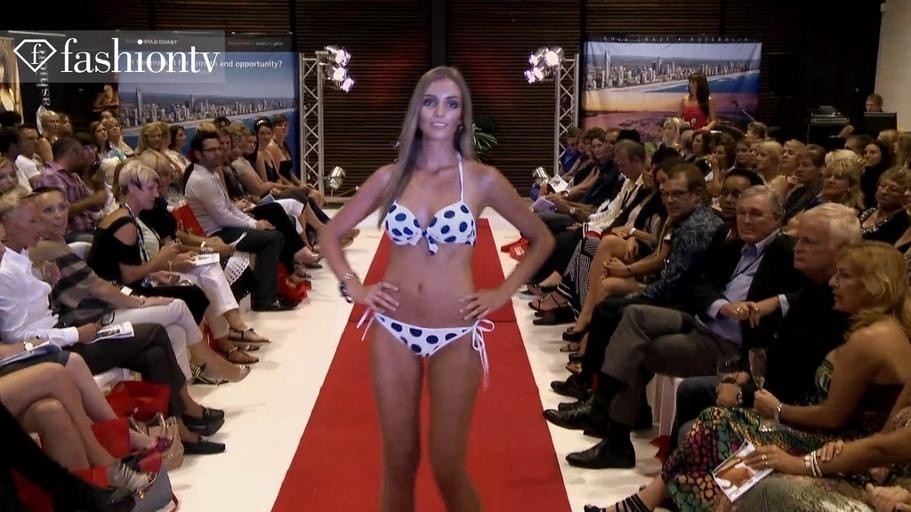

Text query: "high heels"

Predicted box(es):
[561, 323, 589, 340]
[106, 459, 158, 495]
[190, 358, 255, 387]
[295, 251, 323, 268]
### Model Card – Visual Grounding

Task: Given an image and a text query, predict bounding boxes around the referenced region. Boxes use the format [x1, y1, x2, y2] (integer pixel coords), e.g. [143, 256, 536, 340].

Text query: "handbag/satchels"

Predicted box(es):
[129, 413, 185, 472]
[120, 448, 174, 511]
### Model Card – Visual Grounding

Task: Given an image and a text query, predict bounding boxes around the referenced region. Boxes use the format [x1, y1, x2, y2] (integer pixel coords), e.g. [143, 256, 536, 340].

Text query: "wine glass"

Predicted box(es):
[44, 257, 56, 291]
[165, 240, 176, 278]
[748, 348, 777, 431]
[691, 118, 696, 130]
[705, 158, 713, 169]
[717, 359, 739, 384]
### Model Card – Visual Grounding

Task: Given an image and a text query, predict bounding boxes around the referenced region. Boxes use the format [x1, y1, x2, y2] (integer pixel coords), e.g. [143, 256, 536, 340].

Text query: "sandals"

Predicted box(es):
[287, 268, 312, 286]
[528, 291, 569, 312]
[229, 326, 271, 344]
[219, 344, 260, 364]
[583, 494, 654, 512]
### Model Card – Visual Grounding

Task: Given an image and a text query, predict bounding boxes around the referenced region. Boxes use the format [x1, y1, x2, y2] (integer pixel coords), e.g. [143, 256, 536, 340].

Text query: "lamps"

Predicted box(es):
[325, 46, 355, 93]
[325, 166, 346, 191]
[532, 165, 548, 188]
[524, 47, 565, 85]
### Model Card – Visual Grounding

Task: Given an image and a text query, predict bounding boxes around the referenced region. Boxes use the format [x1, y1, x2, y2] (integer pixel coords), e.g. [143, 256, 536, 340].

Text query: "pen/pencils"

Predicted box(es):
[563, 222, 579, 227]
[232, 195, 241, 203]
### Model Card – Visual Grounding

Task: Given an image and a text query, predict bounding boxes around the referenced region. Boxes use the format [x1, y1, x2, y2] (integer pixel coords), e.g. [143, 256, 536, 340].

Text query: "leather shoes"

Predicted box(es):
[533, 305, 576, 327]
[182, 434, 226, 455]
[186, 404, 227, 437]
[552, 374, 654, 431]
[253, 293, 298, 311]
[566, 439, 636, 470]
[542, 402, 610, 438]
[561, 343, 586, 374]
[101, 487, 136, 512]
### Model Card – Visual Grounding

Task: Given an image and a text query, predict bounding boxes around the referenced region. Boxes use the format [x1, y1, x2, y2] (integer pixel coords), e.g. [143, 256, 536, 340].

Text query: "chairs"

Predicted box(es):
[91, 364, 141, 391]
[646, 374, 685, 438]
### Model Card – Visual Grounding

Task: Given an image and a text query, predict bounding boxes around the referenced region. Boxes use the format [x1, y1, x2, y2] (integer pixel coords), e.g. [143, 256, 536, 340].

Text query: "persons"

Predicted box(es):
[320, 65, 557, 511]
[2, 109, 362, 512]
[521, 93, 910, 512]
[677, 72, 716, 132]
[1, 44, 18, 113]
[93, 83, 122, 119]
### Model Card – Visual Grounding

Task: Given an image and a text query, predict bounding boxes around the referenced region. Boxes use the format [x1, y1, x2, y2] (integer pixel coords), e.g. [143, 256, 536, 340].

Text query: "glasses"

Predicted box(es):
[81, 144, 98, 153]
[200, 146, 223, 154]
[877, 183, 904, 195]
[604, 139, 618, 146]
[662, 188, 692, 201]
[786, 236, 835, 250]
